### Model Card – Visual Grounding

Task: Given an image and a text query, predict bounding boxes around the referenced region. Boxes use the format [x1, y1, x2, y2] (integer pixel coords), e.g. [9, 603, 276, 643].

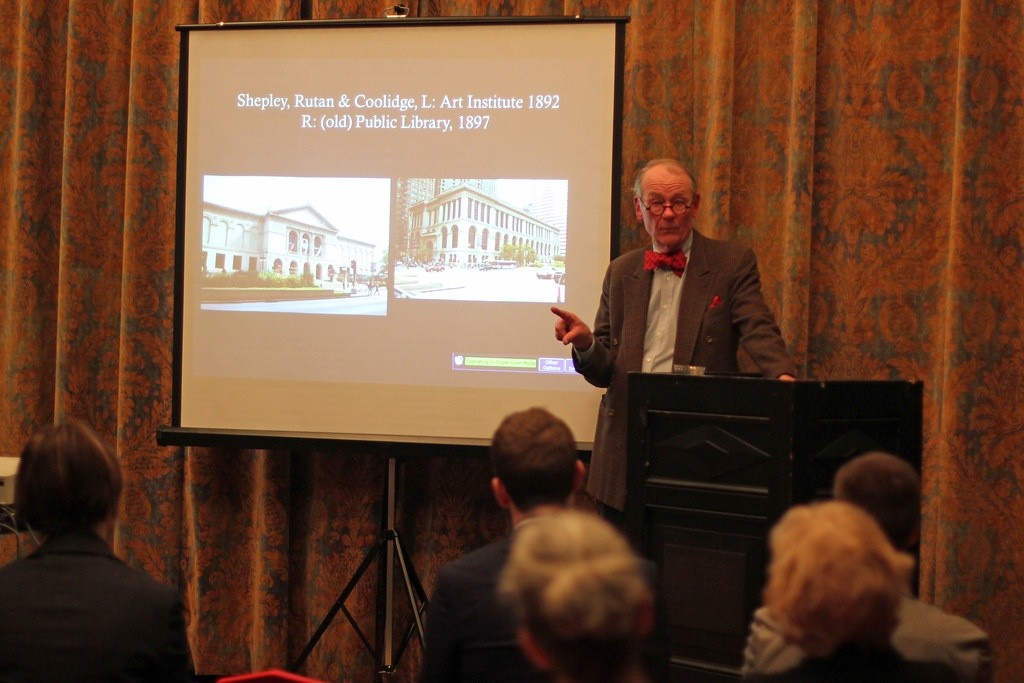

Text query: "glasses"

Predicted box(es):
[635, 192, 696, 217]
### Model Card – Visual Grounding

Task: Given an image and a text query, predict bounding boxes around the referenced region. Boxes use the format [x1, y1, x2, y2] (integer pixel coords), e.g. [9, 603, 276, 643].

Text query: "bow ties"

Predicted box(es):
[643, 248, 688, 277]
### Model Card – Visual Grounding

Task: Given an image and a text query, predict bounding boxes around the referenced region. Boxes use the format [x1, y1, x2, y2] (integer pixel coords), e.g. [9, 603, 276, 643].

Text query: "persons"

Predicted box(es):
[368, 281, 372, 296]
[374, 281, 380, 295]
[0, 417, 195, 683]
[745, 453, 994, 683]
[419, 407, 652, 683]
[746, 499, 956, 683]
[498, 506, 669, 683]
[549, 157, 798, 527]
